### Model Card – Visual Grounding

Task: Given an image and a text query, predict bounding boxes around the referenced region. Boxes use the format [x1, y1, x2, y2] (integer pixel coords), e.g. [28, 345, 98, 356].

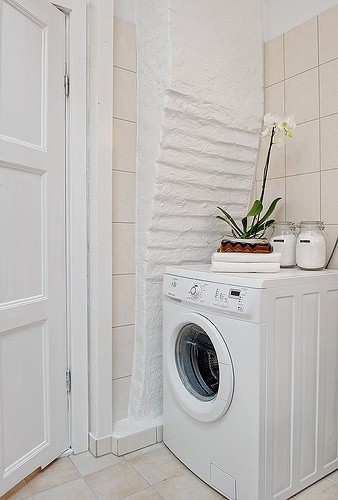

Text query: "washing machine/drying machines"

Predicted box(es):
[161, 261, 338, 500]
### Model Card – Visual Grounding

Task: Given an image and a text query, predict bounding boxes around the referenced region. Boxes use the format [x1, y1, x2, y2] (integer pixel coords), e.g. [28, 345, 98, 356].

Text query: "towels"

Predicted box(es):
[210, 252, 281, 273]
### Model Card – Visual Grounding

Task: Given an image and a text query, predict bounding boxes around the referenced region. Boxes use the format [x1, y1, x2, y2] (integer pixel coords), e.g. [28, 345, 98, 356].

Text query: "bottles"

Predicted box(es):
[296, 220, 328, 269]
[270, 221, 297, 267]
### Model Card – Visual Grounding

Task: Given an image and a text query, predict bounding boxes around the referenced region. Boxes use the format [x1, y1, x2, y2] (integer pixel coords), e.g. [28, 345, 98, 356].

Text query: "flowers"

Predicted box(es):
[216, 112, 297, 239]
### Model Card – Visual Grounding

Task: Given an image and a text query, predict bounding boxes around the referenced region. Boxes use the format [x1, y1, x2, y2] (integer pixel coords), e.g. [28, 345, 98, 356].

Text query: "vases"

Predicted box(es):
[211, 235, 282, 273]
